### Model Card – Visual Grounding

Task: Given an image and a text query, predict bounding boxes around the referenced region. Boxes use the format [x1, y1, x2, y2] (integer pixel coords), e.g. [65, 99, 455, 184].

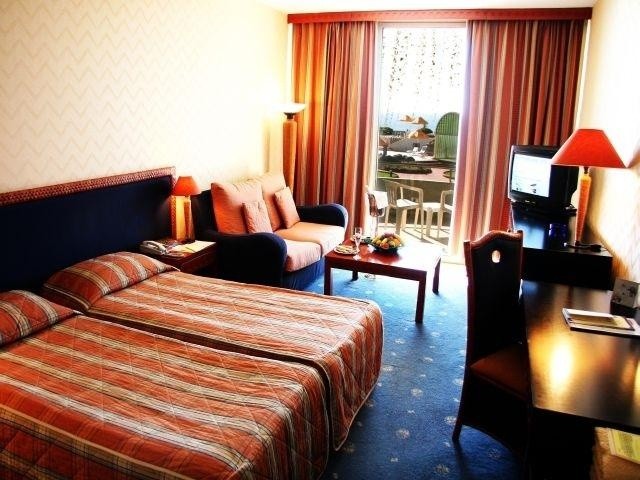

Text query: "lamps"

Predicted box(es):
[550, 128, 627, 250]
[171, 176, 201, 242]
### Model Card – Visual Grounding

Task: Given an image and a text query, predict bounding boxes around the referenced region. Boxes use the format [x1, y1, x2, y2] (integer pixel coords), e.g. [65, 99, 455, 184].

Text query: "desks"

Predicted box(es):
[511, 201, 612, 287]
[522, 280, 640, 429]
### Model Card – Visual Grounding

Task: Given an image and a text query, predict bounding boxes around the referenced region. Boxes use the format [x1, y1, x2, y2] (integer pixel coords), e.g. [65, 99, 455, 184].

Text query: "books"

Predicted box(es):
[561, 307, 640, 336]
[607, 427, 640, 465]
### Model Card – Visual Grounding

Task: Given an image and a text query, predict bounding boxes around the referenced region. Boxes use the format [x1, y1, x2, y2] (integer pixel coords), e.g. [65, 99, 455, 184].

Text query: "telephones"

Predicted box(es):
[140, 240, 168, 256]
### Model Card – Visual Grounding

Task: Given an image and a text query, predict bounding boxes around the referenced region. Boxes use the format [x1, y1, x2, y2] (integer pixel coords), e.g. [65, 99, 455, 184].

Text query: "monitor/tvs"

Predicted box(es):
[507, 144, 579, 218]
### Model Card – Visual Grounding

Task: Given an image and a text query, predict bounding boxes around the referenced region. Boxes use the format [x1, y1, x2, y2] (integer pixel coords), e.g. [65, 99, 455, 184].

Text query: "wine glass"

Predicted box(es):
[354, 227, 362, 260]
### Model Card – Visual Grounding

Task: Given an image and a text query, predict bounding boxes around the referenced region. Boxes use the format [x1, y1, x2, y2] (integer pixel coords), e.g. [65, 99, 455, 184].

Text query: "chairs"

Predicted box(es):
[453, 231, 533, 455]
[385, 179, 423, 238]
[416, 190, 454, 239]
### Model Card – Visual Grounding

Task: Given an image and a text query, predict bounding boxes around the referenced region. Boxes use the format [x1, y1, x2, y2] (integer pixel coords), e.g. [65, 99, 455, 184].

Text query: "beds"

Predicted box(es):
[0, 270, 383, 479]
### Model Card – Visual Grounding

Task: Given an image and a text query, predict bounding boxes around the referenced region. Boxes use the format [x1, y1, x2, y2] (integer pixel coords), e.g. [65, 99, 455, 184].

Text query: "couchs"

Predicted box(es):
[198, 171, 348, 291]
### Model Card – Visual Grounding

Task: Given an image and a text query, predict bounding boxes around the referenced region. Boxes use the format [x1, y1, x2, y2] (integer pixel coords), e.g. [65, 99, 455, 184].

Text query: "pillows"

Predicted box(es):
[262, 171, 286, 229]
[1, 290, 75, 346]
[41, 252, 178, 308]
[244, 199, 272, 236]
[273, 187, 300, 227]
[211, 179, 263, 237]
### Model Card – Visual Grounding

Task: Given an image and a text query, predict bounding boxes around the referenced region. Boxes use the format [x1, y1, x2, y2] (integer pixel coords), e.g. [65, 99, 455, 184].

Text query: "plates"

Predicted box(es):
[371, 236, 402, 253]
[333, 244, 361, 255]
[350, 235, 366, 243]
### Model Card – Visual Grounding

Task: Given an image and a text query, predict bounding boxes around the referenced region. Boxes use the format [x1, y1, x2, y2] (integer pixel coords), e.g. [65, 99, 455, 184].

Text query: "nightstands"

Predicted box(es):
[142, 239, 215, 274]
